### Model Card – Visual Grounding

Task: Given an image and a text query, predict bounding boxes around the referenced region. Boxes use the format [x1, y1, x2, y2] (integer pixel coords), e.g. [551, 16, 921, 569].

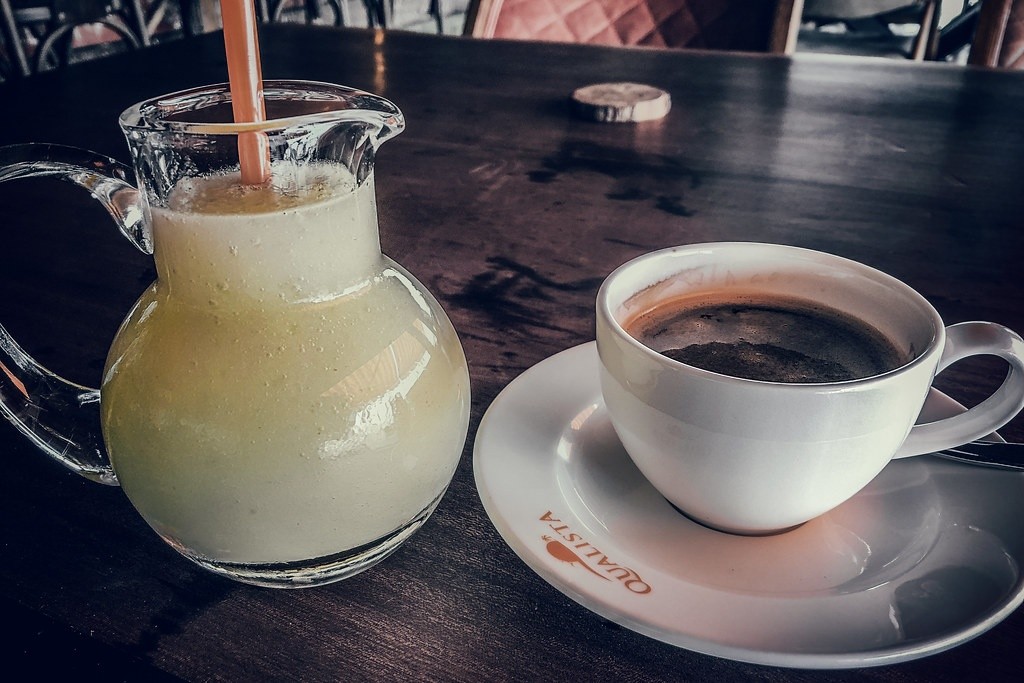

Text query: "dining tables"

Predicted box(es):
[0, 21, 1024, 683]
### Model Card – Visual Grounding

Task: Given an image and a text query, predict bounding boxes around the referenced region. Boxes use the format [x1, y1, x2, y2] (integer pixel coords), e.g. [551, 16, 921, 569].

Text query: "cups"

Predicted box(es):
[597, 241, 1023, 534]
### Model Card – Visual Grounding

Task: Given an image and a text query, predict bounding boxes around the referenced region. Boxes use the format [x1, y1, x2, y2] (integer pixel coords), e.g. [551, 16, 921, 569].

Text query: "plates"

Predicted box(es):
[473, 339, 1024, 669]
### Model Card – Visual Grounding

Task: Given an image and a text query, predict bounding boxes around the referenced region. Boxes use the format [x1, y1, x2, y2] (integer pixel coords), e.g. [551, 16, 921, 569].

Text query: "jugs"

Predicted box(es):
[0, 80, 471, 589]
